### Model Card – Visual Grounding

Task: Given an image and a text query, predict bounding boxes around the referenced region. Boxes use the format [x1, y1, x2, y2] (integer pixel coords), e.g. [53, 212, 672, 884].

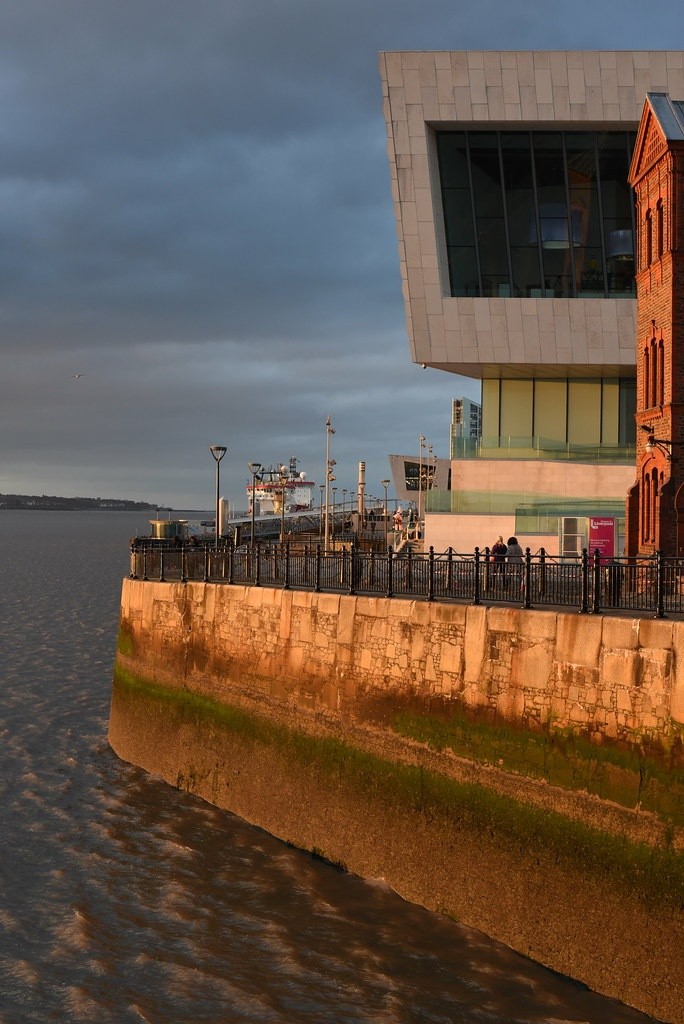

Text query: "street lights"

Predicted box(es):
[209, 445, 228, 553]
[350, 491, 354, 534]
[340, 490, 348, 535]
[331, 488, 338, 535]
[277, 475, 290, 545]
[364, 494, 383, 524]
[382, 479, 390, 553]
[359, 482, 364, 539]
[325, 428, 333, 558]
[318, 485, 325, 541]
[248, 463, 262, 550]
[419, 433, 437, 520]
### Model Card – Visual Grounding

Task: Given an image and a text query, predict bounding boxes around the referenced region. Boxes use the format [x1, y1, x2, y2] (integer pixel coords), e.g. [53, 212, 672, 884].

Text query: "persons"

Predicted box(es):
[490, 536, 508, 588]
[370, 518, 376, 534]
[363, 518, 367, 530]
[173, 536, 183, 569]
[368, 509, 375, 520]
[503, 537, 525, 590]
[187, 536, 201, 569]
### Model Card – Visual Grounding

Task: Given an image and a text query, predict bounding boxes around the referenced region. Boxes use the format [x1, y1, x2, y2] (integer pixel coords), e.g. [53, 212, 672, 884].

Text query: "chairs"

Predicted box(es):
[234, 545, 252, 554]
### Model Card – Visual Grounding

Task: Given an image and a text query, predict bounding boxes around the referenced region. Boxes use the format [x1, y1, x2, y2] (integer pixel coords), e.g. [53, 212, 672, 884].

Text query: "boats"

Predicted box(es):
[186, 456, 381, 547]
